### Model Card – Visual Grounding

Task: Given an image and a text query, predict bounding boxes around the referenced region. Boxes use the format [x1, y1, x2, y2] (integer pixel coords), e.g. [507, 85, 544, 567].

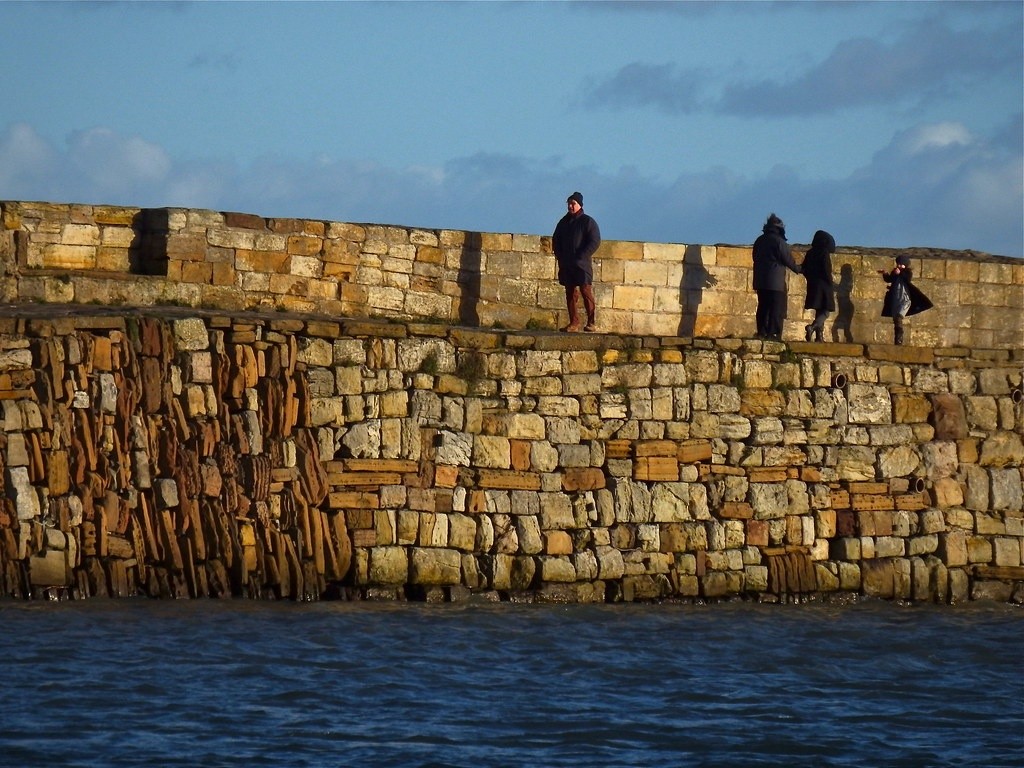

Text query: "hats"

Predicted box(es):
[568, 192, 583, 206]
[896, 256, 909, 266]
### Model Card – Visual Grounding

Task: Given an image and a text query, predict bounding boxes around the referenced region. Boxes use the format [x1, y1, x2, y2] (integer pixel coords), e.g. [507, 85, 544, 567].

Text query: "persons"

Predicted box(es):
[881, 255, 914, 345]
[801, 230, 835, 343]
[752, 214, 802, 343]
[552, 192, 601, 333]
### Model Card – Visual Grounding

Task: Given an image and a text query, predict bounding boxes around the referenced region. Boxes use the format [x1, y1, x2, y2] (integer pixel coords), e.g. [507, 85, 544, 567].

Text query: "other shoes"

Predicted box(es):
[559, 324, 578, 332]
[815, 327, 825, 342]
[805, 324, 815, 341]
[584, 323, 595, 331]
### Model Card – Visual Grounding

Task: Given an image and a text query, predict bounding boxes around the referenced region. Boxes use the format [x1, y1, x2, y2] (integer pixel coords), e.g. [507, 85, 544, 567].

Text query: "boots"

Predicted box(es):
[895, 327, 903, 344]
